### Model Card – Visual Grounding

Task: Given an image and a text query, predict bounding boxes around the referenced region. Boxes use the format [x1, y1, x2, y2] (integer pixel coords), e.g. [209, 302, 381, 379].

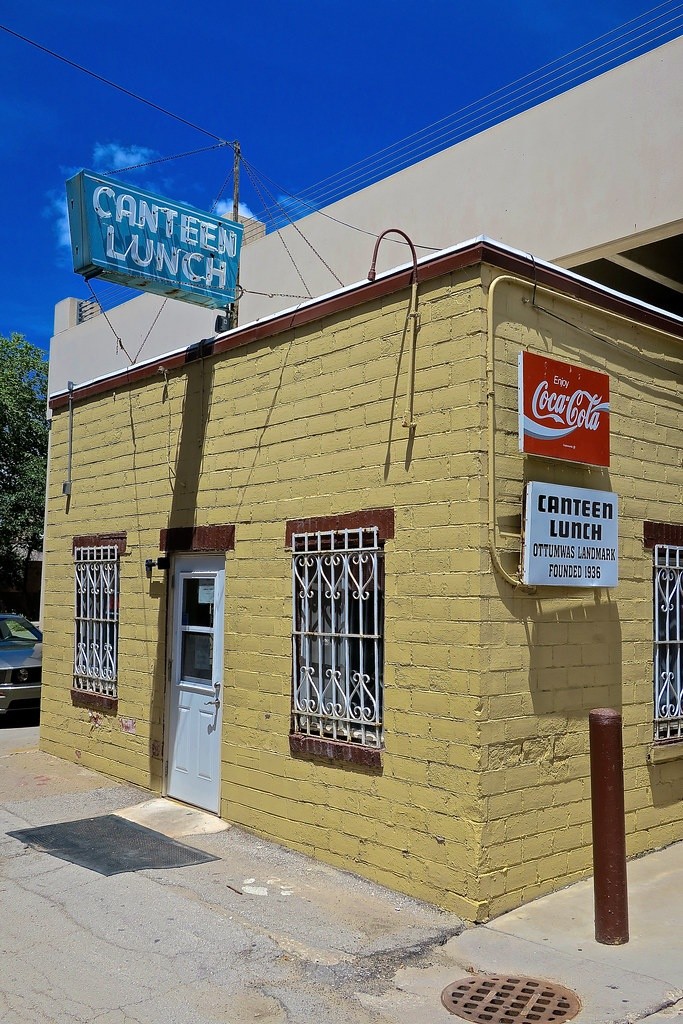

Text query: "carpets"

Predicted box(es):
[4, 815, 222, 877]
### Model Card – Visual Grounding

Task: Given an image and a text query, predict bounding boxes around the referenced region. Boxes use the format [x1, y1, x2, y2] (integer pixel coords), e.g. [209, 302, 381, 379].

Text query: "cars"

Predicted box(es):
[0, 614, 43, 714]
